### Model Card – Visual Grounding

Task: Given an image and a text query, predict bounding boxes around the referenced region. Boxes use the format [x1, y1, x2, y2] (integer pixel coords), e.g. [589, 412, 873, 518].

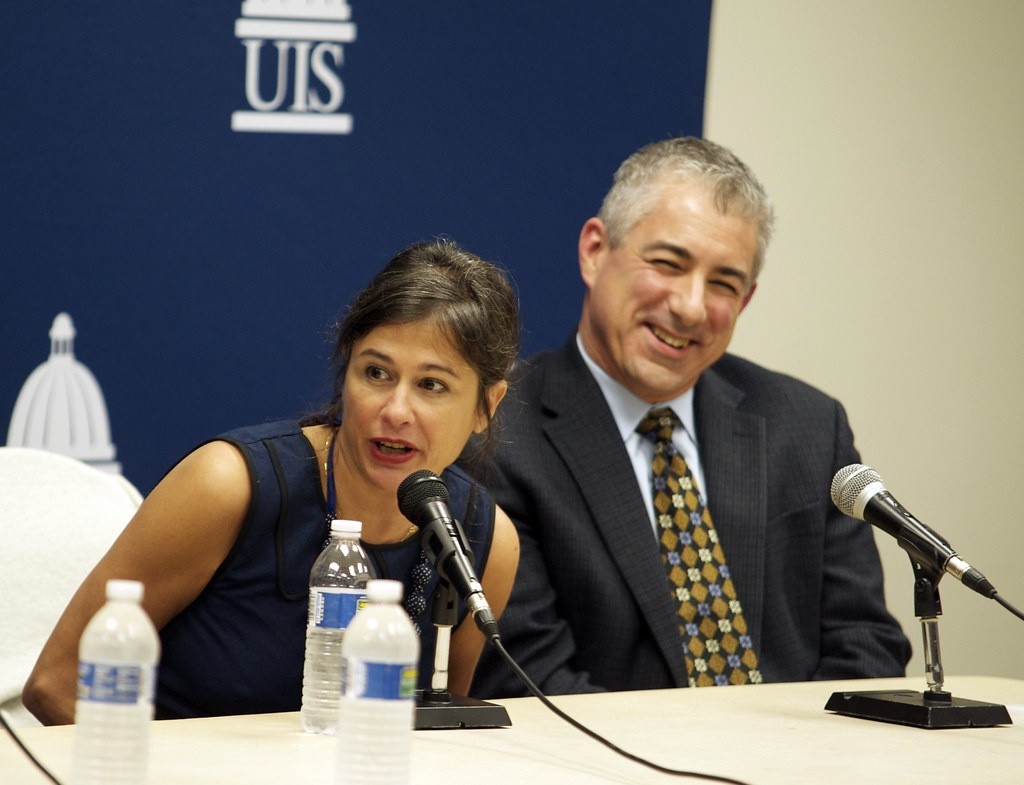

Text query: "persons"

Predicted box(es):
[459, 135, 913, 699]
[21, 239, 520, 728]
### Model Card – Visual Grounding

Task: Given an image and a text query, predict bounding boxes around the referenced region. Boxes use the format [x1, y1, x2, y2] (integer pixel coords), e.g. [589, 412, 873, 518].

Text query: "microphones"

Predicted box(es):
[830, 463, 996, 600]
[395, 469, 500, 646]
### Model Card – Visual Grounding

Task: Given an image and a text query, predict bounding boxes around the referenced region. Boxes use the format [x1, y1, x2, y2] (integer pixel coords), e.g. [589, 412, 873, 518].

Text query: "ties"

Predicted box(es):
[634, 408, 763, 689]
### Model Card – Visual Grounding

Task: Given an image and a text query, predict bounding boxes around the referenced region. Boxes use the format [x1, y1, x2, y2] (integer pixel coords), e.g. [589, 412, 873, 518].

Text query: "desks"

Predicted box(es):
[1, 675, 1024, 784]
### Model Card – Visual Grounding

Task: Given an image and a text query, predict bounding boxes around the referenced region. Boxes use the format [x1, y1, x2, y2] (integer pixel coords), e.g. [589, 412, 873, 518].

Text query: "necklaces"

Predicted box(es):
[324, 431, 419, 542]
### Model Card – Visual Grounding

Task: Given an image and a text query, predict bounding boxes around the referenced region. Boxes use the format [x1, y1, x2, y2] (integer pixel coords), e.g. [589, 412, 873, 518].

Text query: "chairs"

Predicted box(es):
[0, 445, 144, 726]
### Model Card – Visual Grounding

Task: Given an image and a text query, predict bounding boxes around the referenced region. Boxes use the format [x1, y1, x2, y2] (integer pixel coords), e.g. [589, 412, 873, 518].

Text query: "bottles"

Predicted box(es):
[72, 579, 159, 785]
[330, 579, 418, 785]
[300, 518, 374, 736]
